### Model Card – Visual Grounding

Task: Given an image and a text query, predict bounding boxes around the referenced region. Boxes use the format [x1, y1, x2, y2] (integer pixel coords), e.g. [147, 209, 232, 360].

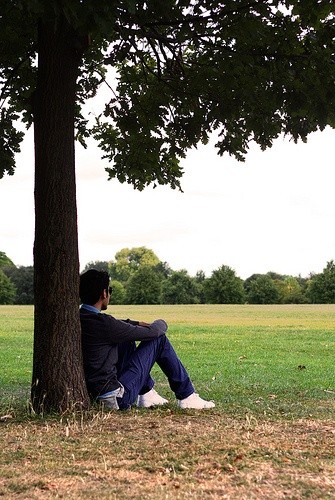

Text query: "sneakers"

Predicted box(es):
[178, 392, 215, 409]
[137, 388, 168, 408]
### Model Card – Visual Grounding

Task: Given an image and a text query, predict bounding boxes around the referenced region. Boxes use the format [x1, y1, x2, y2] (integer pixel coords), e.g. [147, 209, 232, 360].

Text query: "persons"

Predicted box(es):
[79, 269, 215, 411]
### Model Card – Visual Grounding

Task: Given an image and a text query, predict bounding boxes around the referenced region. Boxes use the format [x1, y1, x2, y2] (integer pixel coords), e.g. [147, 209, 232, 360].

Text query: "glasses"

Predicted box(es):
[108, 287, 112, 295]
